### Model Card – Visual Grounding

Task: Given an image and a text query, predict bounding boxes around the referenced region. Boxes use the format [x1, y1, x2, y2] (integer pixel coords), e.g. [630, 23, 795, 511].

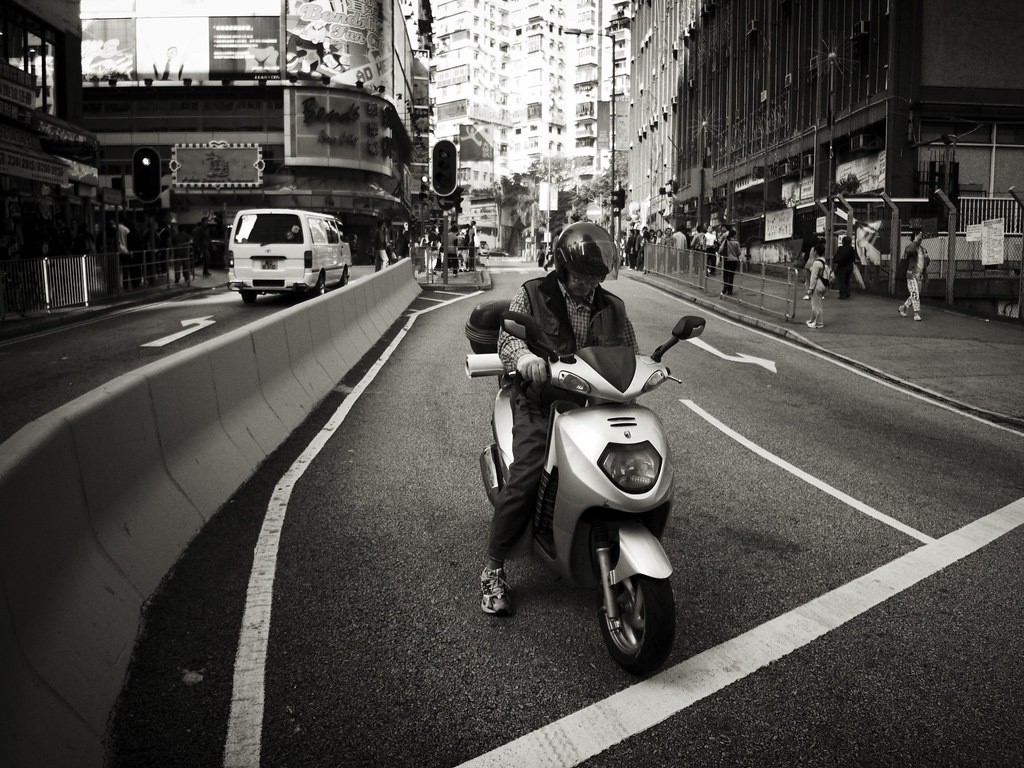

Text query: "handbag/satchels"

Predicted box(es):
[719, 240, 728, 257]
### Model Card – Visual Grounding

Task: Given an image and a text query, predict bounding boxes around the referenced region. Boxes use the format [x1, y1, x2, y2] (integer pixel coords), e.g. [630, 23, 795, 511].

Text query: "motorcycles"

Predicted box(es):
[462, 298, 707, 675]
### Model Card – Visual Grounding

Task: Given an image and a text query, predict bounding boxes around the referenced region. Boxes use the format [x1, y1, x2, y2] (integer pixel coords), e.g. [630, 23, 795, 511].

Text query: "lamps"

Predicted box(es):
[376, 85, 385, 92]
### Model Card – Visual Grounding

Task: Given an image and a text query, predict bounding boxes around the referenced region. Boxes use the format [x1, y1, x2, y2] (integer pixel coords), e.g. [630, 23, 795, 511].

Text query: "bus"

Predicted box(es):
[476, 240, 491, 257]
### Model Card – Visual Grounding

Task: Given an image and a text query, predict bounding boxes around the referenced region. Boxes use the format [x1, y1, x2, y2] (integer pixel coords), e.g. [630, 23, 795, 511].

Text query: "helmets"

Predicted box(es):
[555, 222, 620, 283]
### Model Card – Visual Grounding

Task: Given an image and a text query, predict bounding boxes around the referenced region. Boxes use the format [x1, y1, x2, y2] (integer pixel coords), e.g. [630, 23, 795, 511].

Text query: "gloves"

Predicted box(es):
[517, 354, 547, 383]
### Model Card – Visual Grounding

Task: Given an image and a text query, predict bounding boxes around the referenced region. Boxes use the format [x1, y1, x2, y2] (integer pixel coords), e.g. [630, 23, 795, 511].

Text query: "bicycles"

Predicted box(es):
[0, 270, 46, 318]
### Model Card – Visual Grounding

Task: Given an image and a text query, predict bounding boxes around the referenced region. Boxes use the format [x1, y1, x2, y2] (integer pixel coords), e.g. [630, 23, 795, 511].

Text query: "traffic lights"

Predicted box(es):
[435, 144, 454, 192]
[137, 150, 156, 201]
[455, 186, 464, 214]
[611, 190, 621, 217]
[421, 175, 430, 203]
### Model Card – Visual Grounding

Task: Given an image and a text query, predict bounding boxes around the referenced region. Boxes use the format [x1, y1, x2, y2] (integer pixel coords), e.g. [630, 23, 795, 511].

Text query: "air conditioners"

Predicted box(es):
[746, 20, 758, 37]
[720, 188, 727, 197]
[672, 20, 696, 52]
[851, 19, 870, 41]
[802, 154, 814, 170]
[638, 79, 694, 139]
[778, 163, 791, 177]
[851, 134, 879, 152]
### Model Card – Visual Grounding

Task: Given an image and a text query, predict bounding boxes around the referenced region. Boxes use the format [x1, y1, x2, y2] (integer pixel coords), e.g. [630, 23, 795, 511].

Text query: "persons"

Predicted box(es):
[0, 214, 193, 309]
[544, 255, 554, 272]
[397, 221, 475, 278]
[801, 230, 827, 300]
[538, 248, 544, 267]
[718, 229, 741, 295]
[806, 244, 830, 329]
[191, 217, 211, 275]
[375, 220, 389, 269]
[898, 229, 930, 321]
[624, 224, 732, 277]
[481, 222, 639, 614]
[832, 236, 855, 299]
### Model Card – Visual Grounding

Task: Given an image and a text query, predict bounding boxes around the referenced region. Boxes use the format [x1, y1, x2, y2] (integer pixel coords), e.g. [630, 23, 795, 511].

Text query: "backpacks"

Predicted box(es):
[816, 259, 830, 287]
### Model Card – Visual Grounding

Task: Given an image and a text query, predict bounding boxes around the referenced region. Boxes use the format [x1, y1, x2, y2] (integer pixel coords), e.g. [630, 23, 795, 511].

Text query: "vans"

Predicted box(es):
[225, 207, 357, 306]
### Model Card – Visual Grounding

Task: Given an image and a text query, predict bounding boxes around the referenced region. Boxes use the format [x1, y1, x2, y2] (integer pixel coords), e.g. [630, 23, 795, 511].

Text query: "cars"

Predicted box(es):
[489, 249, 510, 257]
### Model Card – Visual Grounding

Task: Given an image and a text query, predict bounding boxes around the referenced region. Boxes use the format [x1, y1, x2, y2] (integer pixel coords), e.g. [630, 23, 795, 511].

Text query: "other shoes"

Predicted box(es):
[803, 295, 809, 300]
[822, 297, 824, 299]
[454, 274, 457, 277]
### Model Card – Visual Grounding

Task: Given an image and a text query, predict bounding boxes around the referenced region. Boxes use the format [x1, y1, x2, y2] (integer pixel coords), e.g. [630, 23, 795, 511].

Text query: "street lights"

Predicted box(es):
[565, 26, 620, 281]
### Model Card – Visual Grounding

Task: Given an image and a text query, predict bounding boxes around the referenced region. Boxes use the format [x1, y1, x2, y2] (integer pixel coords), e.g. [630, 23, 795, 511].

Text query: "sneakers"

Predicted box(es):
[914, 315, 922, 320]
[898, 306, 907, 316]
[806, 320, 824, 328]
[480, 567, 511, 613]
